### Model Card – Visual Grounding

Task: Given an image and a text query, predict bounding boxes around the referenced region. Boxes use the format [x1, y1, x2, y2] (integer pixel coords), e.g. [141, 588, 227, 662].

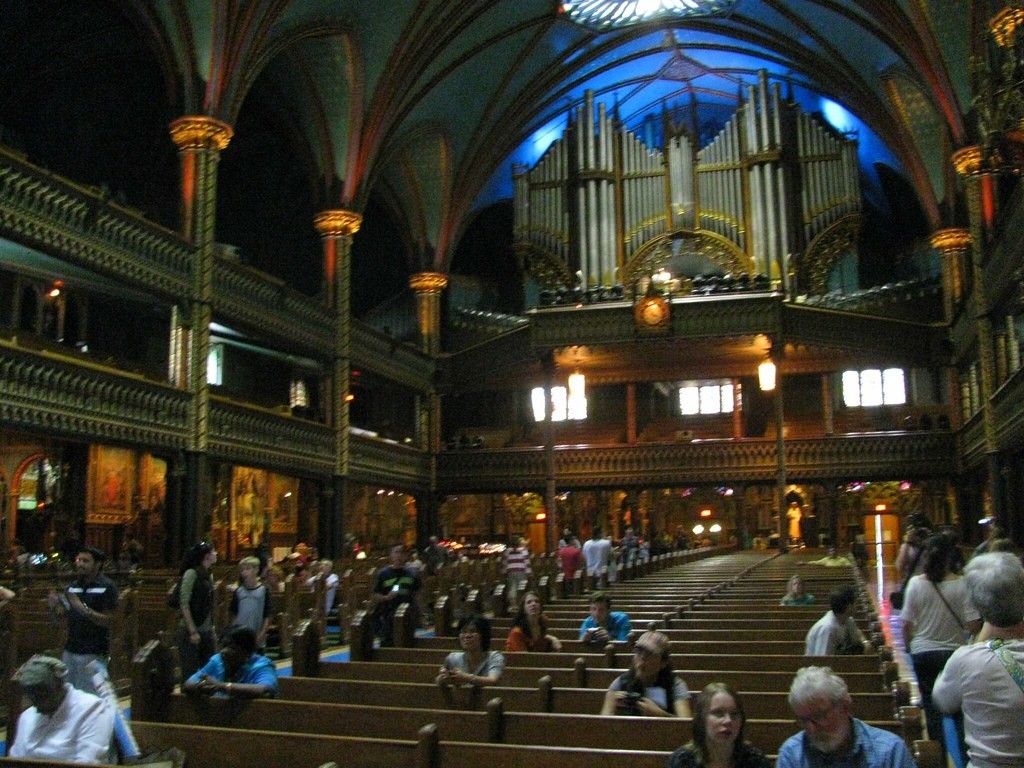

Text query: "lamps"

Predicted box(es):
[757, 345, 780, 391]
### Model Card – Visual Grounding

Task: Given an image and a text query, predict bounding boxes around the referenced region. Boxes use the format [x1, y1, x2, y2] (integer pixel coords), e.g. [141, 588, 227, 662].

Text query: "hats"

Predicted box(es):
[635, 632, 670, 655]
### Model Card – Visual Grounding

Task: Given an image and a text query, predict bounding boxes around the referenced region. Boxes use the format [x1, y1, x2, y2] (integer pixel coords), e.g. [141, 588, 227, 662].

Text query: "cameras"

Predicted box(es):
[914, 511, 928, 538]
[587, 627, 599, 635]
[624, 692, 641, 705]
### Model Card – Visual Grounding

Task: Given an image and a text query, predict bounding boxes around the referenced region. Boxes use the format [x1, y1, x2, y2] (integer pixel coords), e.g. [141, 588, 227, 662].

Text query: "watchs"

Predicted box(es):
[225, 682, 233, 692]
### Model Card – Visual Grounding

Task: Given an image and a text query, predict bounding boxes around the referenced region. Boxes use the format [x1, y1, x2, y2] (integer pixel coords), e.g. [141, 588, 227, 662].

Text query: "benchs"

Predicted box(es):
[47, 546, 944, 768]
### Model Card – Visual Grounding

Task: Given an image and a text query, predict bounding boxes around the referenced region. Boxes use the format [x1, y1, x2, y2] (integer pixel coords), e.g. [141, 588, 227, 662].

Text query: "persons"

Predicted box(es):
[6, 654, 118, 765]
[578, 590, 635, 643]
[778, 575, 816, 606]
[445, 432, 485, 452]
[902, 411, 953, 431]
[555, 525, 781, 598]
[0, 537, 496, 699]
[601, 631, 693, 718]
[665, 682, 775, 768]
[804, 586, 875, 655]
[500, 534, 533, 605]
[776, 664, 918, 768]
[892, 520, 1024, 768]
[797, 545, 853, 567]
[787, 501, 802, 538]
[432, 612, 506, 710]
[504, 591, 563, 653]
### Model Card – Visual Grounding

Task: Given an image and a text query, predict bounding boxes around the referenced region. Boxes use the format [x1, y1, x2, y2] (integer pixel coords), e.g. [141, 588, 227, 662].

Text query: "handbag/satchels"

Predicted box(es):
[891, 591, 904, 609]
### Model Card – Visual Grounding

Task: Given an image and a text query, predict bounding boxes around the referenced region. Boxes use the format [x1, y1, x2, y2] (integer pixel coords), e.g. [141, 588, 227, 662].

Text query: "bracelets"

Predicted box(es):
[0, 585, 4, 590]
[861, 640, 869, 644]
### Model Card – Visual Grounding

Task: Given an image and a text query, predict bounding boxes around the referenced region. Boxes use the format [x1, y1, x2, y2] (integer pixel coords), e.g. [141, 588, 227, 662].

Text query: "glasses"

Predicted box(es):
[795, 697, 843, 729]
